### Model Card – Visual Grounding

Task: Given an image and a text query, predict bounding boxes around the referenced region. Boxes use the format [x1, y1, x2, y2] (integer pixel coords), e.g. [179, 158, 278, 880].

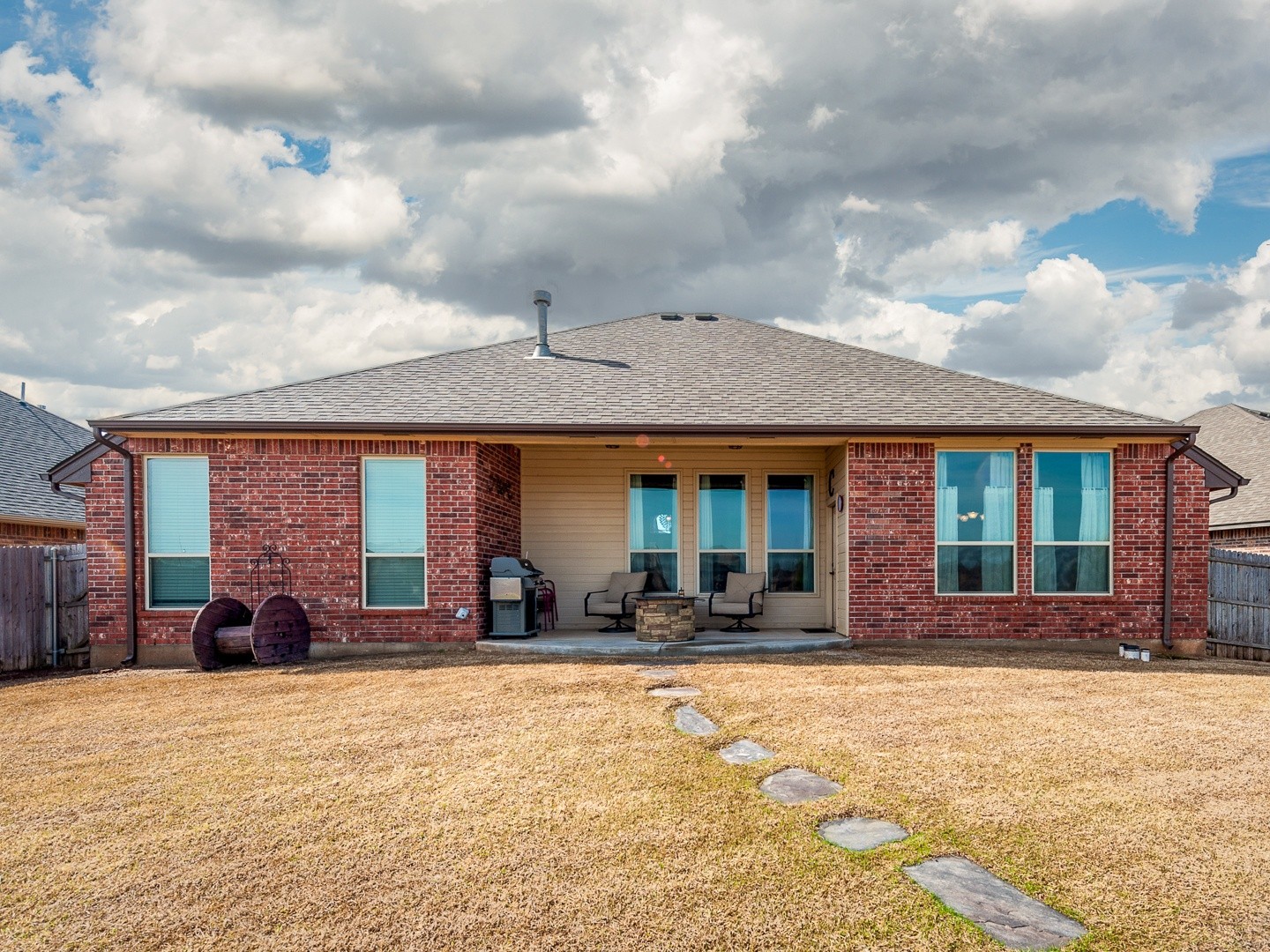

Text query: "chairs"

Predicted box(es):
[584, 572, 647, 633]
[708, 572, 768, 633]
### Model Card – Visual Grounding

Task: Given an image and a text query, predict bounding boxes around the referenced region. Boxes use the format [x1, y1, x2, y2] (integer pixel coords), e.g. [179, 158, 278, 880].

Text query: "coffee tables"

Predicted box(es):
[631, 595, 699, 642]
